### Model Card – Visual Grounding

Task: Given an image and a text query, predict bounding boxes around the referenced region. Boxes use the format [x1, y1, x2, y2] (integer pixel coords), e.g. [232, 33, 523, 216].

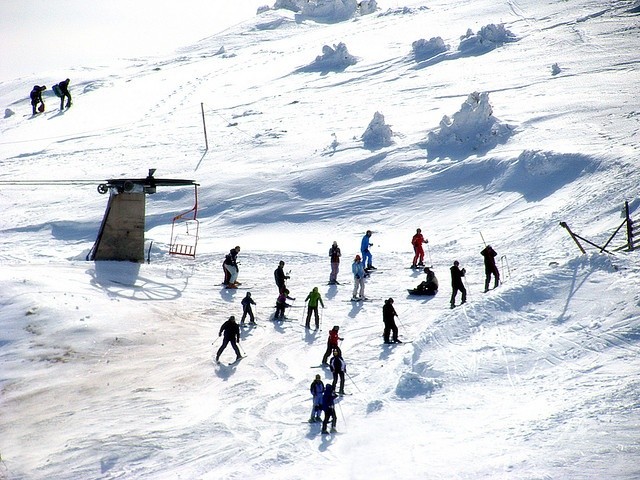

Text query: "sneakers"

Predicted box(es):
[385, 340, 392, 344]
[393, 339, 401, 343]
[316, 418, 322, 422]
[311, 418, 317, 422]
[236, 355, 242, 360]
[322, 431, 328, 434]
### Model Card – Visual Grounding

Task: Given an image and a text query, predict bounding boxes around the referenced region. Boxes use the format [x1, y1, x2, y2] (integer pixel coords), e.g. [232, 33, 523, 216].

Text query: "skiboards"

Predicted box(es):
[368, 269, 391, 274]
[405, 263, 433, 269]
[214, 282, 255, 291]
[275, 306, 306, 308]
[335, 392, 352, 397]
[213, 356, 247, 365]
[266, 318, 298, 322]
[342, 299, 382, 302]
[384, 341, 412, 345]
[311, 366, 326, 368]
[239, 324, 266, 328]
[327, 282, 351, 286]
[302, 421, 330, 423]
[300, 325, 322, 331]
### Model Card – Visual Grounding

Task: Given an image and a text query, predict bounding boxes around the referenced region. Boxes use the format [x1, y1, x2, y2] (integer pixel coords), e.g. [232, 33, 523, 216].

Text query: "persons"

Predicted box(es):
[410, 229, 429, 269]
[274, 260, 295, 307]
[328, 240, 343, 285]
[304, 286, 325, 329]
[274, 289, 291, 320]
[329, 348, 347, 394]
[321, 325, 344, 365]
[351, 254, 369, 302]
[479, 244, 500, 293]
[30, 85, 46, 115]
[309, 373, 324, 423]
[407, 267, 439, 296]
[223, 245, 241, 290]
[215, 315, 242, 362]
[382, 297, 402, 344]
[240, 291, 256, 324]
[360, 230, 378, 270]
[321, 383, 337, 435]
[449, 260, 466, 309]
[52, 78, 71, 110]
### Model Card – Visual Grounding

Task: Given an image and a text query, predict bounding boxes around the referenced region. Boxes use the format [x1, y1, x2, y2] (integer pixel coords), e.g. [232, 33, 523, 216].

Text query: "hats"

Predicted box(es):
[355, 255, 360, 260]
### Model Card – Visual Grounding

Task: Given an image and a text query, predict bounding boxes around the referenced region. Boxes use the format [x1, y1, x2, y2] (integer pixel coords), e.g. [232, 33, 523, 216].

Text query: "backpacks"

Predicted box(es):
[52, 84, 64, 97]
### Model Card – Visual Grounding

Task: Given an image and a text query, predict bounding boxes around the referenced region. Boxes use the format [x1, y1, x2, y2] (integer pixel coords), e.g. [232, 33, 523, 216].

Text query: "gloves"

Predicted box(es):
[356, 273, 360, 279]
[364, 274, 367, 277]
